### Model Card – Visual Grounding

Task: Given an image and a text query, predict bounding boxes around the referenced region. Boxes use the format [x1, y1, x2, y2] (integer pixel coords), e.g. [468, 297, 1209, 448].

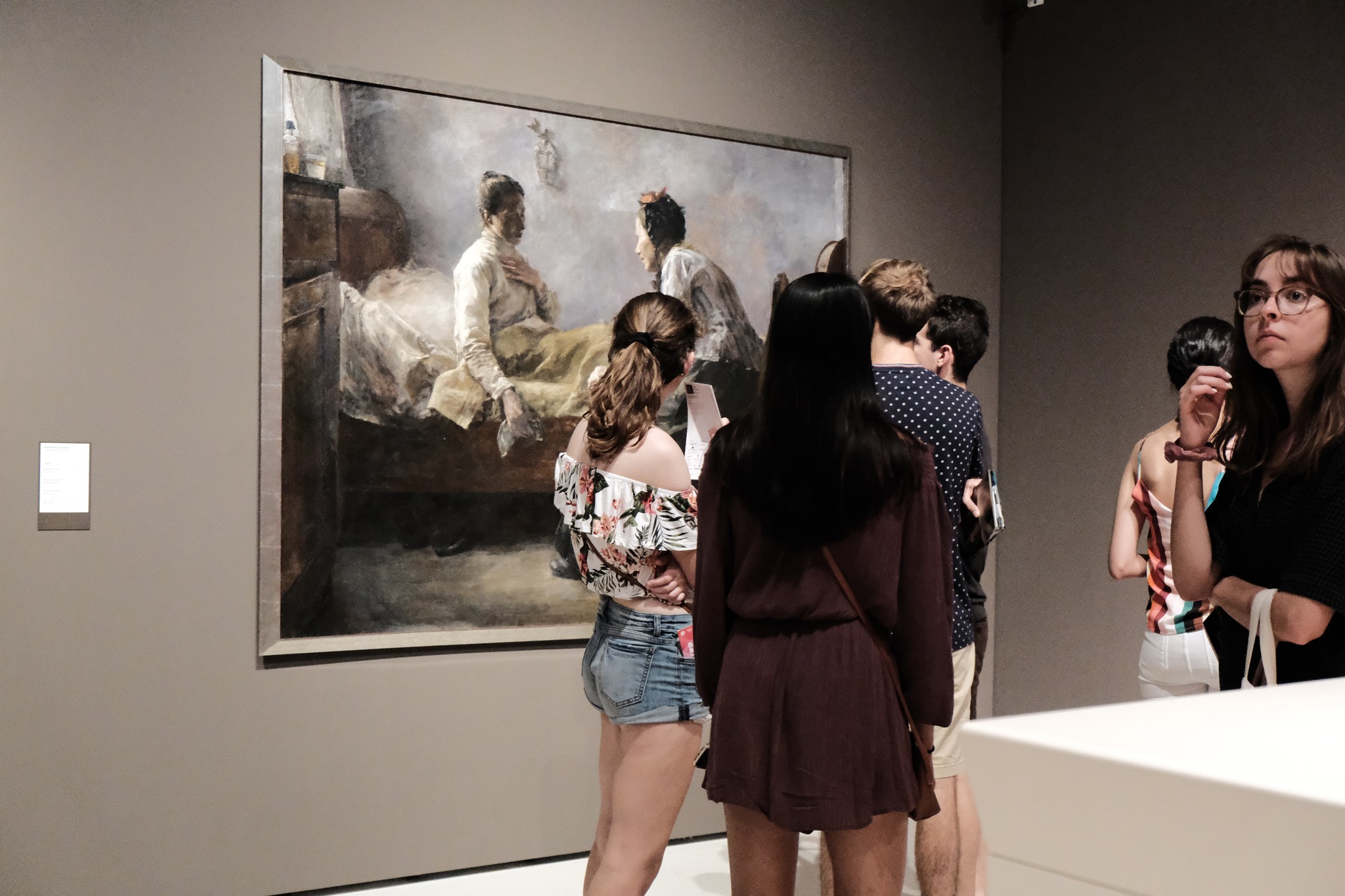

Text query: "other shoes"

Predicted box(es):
[399, 521, 477, 555]
[550, 557, 583, 581]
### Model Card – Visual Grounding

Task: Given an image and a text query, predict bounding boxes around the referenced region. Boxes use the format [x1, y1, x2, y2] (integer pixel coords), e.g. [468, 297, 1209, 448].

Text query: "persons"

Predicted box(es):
[857, 258, 983, 896]
[550, 186, 766, 580]
[556, 292, 701, 896]
[1163, 232, 1345, 691]
[912, 294, 992, 721]
[1109, 315, 1249, 701]
[452, 171, 558, 440]
[693, 271, 953, 896]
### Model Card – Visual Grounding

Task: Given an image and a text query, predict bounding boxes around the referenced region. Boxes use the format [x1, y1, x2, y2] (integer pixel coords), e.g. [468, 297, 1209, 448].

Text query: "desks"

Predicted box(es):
[954, 674, 1345, 896]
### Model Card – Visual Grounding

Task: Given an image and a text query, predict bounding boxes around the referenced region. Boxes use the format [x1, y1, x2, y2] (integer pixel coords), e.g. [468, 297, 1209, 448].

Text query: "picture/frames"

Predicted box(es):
[258, 52, 854, 660]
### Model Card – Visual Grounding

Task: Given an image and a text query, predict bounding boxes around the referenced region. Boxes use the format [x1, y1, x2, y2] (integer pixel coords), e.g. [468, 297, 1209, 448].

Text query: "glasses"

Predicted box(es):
[1233, 286, 1335, 317]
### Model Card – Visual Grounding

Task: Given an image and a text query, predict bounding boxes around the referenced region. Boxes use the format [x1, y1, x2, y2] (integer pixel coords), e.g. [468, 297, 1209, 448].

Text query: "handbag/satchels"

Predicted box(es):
[1240, 587, 1280, 687]
[908, 728, 941, 821]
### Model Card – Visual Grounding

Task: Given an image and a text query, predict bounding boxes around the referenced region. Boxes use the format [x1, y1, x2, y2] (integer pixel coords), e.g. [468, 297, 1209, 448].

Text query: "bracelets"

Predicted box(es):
[1163, 437, 1218, 463]
